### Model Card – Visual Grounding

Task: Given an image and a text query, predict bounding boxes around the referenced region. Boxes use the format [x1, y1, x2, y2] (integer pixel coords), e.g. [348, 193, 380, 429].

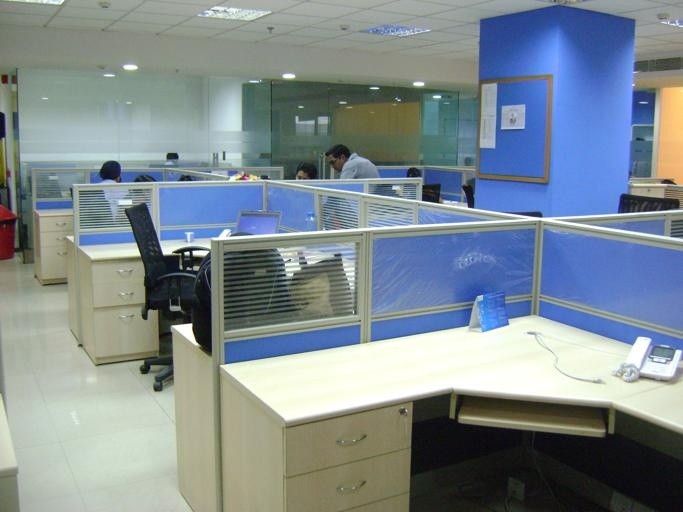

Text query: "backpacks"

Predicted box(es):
[191, 231, 292, 351]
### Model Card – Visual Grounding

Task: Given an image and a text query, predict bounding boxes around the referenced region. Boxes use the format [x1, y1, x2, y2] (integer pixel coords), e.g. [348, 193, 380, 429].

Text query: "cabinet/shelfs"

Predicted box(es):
[34, 208, 74, 285]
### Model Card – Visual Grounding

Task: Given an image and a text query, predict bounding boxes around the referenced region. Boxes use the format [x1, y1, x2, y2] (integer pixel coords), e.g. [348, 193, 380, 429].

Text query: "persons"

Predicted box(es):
[324, 143, 381, 191]
[114, 174, 153, 218]
[293, 161, 317, 181]
[91, 161, 128, 221]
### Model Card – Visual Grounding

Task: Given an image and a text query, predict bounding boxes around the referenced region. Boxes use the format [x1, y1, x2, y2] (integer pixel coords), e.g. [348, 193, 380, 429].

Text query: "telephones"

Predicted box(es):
[219, 229, 232, 237]
[611, 336, 682, 381]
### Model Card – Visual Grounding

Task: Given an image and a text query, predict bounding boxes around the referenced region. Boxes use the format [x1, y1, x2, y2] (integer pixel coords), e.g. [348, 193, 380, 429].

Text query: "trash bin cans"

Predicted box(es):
[0, 204, 17, 260]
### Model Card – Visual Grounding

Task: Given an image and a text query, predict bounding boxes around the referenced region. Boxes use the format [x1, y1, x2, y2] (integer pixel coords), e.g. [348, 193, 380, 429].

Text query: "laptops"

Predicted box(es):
[236, 209, 282, 237]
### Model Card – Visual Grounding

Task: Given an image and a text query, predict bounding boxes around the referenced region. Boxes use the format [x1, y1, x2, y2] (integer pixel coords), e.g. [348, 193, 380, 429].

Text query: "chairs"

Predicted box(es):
[125, 201, 210, 392]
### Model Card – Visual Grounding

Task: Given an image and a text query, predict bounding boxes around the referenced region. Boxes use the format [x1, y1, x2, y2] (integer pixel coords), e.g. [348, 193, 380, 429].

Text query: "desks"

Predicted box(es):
[78, 235, 356, 511]
[220, 313, 683, 510]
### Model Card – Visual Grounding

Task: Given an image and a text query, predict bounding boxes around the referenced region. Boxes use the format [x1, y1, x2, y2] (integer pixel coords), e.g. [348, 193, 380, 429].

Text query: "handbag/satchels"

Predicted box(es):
[290, 250, 354, 319]
